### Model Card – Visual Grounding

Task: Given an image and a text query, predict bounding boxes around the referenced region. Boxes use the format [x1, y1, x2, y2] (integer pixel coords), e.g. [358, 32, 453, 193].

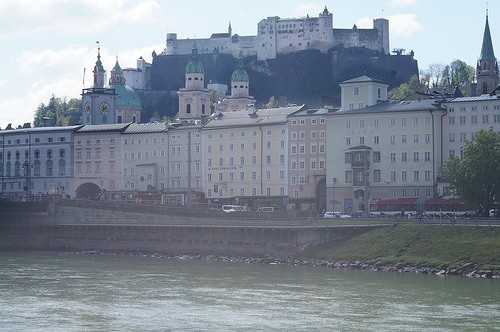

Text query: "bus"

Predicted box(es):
[369, 198, 478, 217]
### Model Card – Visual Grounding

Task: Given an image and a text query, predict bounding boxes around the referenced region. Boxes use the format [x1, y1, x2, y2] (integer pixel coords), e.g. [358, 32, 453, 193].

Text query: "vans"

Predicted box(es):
[221, 205, 248, 212]
[324, 212, 342, 218]
[254, 207, 279, 212]
